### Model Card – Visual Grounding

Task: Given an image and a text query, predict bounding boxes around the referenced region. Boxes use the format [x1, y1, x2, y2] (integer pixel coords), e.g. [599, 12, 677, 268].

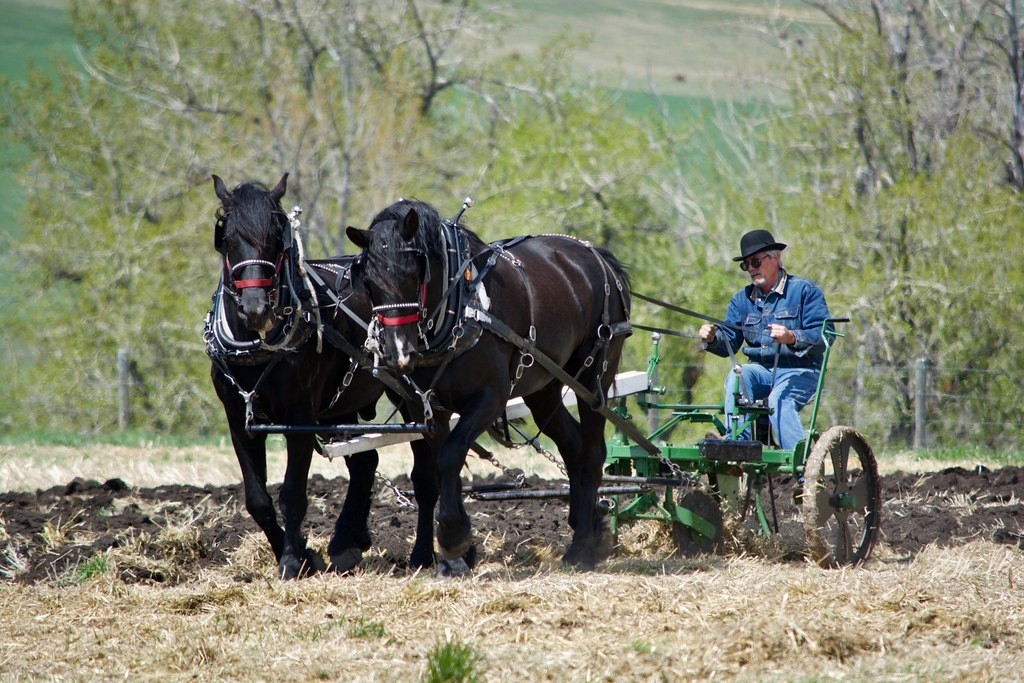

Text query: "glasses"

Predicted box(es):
[740, 254, 769, 271]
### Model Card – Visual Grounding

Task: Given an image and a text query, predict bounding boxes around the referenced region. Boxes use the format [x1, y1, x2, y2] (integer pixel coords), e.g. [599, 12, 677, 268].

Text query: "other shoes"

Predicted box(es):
[791, 477, 823, 493]
[704, 431, 745, 477]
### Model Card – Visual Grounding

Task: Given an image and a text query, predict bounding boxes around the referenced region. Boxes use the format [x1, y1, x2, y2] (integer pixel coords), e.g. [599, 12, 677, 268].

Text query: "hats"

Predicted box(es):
[732, 230, 787, 262]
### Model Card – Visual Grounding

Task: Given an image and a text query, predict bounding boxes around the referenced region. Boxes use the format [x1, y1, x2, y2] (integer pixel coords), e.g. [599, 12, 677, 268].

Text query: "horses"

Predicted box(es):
[346, 200, 634, 575]
[209, 173, 441, 580]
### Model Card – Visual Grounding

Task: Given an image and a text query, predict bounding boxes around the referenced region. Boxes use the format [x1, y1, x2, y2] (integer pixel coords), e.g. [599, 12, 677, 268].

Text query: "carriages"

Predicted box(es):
[204, 172, 881, 570]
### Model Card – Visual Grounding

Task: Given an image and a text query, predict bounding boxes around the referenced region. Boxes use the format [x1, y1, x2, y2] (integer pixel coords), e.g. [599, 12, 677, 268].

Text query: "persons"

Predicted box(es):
[700, 230, 836, 499]
